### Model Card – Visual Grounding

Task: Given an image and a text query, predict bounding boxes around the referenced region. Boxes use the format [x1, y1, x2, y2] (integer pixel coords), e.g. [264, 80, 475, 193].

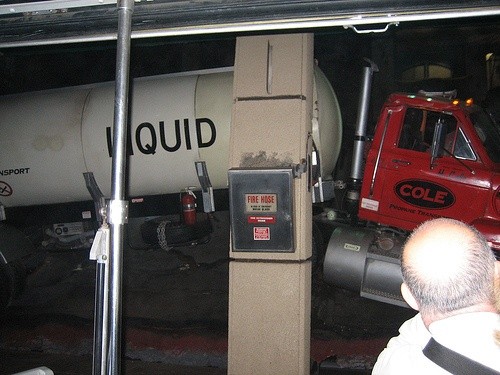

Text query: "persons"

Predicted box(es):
[370, 218, 500, 375]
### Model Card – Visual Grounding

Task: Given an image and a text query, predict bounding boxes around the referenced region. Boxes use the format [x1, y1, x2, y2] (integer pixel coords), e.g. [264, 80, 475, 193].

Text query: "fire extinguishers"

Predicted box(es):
[178, 185, 198, 227]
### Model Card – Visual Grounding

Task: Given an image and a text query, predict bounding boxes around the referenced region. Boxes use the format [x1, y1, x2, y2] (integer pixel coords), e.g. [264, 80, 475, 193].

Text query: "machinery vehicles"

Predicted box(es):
[0, 59, 500, 309]
